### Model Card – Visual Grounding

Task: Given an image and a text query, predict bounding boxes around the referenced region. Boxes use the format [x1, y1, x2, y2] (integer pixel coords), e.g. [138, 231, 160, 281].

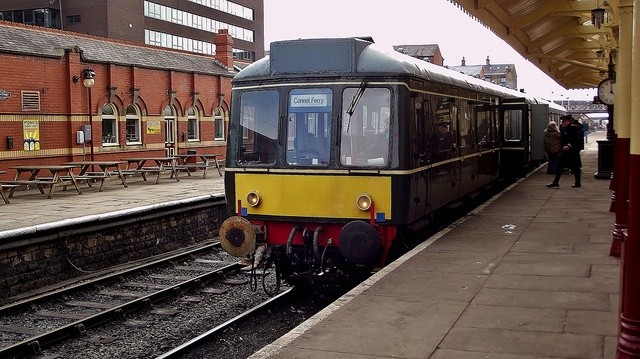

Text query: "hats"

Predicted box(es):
[560, 113, 572, 120]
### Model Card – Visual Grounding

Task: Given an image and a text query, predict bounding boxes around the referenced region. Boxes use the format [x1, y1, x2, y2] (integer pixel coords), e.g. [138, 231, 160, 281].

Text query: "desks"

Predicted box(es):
[120, 157, 181, 185]
[8, 166, 83, 199]
[60, 161, 128, 192]
[0, 171, 10, 204]
[169, 154, 224, 179]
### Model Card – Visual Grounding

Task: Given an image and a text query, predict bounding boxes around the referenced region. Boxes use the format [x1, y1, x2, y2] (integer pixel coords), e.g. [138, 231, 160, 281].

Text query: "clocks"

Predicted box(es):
[592, 51, 616, 179]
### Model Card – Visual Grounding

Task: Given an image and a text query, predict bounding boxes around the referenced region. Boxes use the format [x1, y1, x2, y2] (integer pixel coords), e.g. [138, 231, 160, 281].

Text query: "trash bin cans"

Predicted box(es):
[187, 150, 196, 172]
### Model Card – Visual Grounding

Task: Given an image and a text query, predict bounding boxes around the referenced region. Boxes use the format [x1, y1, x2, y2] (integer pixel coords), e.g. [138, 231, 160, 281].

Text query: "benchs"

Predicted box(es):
[37, 175, 109, 180]
[84, 169, 161, 175]
[1, 185, 20, 198]
[0, 180, 60, 185]
[141, 165, 206, 170]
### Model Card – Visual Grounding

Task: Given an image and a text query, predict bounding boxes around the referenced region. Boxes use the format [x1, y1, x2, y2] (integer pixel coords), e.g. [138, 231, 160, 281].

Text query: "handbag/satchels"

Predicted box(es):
[555, 144, 580, 167]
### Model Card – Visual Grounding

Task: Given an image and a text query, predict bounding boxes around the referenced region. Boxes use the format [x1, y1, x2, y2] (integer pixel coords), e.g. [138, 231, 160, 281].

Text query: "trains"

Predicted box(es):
[212, 35, 567, 295]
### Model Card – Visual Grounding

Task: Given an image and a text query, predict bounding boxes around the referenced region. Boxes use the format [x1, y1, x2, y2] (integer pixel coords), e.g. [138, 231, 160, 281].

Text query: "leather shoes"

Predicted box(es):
[572, 183, 581, 187]
[546, 182, 559, 187]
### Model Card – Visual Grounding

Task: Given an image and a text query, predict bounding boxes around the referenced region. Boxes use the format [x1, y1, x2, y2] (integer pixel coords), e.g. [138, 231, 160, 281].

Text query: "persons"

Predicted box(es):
[433, 120, 452, 157]
[579, 120, 588, 143]
[253, 124, 274, 165]
[546, 114, 584, 187]
[354, 127, 379, 161]
[543, 120, 559, 173]
[301, 134, 321, 157]
[572, 116, 584, 174]
